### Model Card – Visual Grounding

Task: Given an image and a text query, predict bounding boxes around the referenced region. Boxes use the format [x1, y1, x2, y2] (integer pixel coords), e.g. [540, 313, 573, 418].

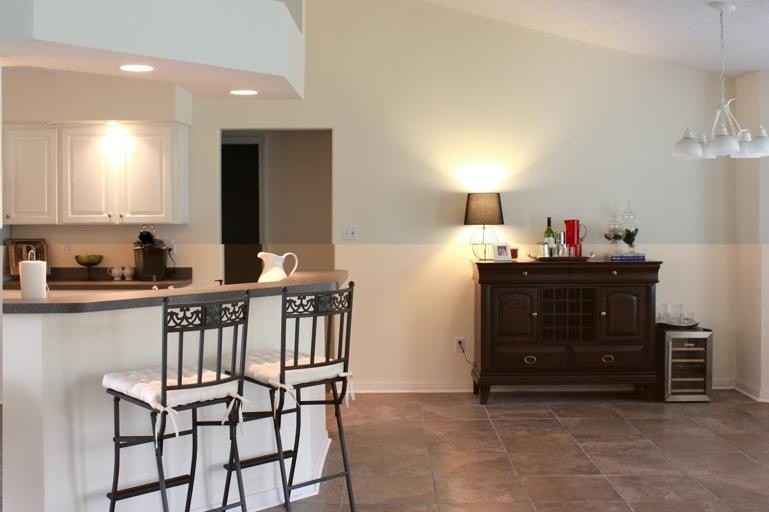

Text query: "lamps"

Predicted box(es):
[464, 188, 505, 262]
[669, 1, 769, 164]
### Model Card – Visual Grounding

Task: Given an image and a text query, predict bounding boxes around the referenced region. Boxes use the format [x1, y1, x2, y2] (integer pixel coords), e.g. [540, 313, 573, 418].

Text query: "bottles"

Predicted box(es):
[543, 217, 555, 245]
[608, 201, 639, 256]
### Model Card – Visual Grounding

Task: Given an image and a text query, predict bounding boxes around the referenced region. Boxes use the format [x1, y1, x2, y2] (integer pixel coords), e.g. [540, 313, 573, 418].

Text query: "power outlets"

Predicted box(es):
[166, 243, 176, 254]
[454, 335, 466, 355]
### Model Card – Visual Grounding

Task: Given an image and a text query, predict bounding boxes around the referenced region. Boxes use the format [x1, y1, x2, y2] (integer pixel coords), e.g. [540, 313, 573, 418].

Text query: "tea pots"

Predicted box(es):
[564, 219, 587, 245]
[257, 251, 299, 283]
[106, 264, 136, 281]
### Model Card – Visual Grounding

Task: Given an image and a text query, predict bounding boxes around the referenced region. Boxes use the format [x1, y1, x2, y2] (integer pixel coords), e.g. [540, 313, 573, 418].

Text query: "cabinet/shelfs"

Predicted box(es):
[656, 326, 713, 404]
[2, 119, 59, 226]
[60, 117, 190, 228]
[470, 255, 664, 406]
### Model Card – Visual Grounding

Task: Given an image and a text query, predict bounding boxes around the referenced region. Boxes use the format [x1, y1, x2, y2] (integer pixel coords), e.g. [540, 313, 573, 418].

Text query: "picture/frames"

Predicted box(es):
[491, 242, 514, 262]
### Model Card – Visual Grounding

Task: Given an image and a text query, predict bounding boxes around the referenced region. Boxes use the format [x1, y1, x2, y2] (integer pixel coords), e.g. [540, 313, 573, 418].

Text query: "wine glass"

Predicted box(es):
[75, 254, 103, 281]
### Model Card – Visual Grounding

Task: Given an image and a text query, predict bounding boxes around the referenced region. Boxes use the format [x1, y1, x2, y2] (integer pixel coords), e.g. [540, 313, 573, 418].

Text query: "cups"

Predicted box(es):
[535, 231, 582, 257]
[656, 303, 696, 326]
[511, 249, 518, 259]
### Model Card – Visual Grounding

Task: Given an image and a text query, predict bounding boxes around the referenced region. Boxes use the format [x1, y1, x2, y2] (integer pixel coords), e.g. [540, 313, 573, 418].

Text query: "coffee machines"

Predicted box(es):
[132, 234, 168, 281]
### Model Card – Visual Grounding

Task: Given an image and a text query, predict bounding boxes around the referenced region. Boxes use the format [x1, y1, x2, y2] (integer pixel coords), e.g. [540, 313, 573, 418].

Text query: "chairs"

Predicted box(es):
[98, 289, 251, 512]
[223, 279, 363, 511]
